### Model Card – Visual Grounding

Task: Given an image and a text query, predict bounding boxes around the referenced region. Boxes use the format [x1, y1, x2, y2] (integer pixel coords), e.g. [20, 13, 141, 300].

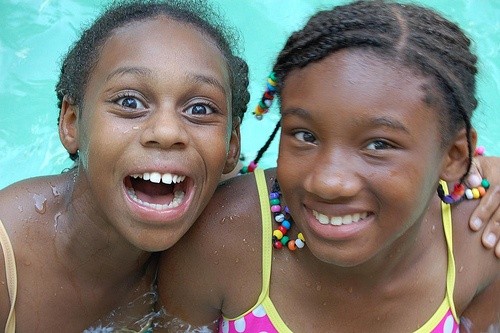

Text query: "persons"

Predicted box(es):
[156, 0, 499, 333]
[0, 0, 498, 331]
